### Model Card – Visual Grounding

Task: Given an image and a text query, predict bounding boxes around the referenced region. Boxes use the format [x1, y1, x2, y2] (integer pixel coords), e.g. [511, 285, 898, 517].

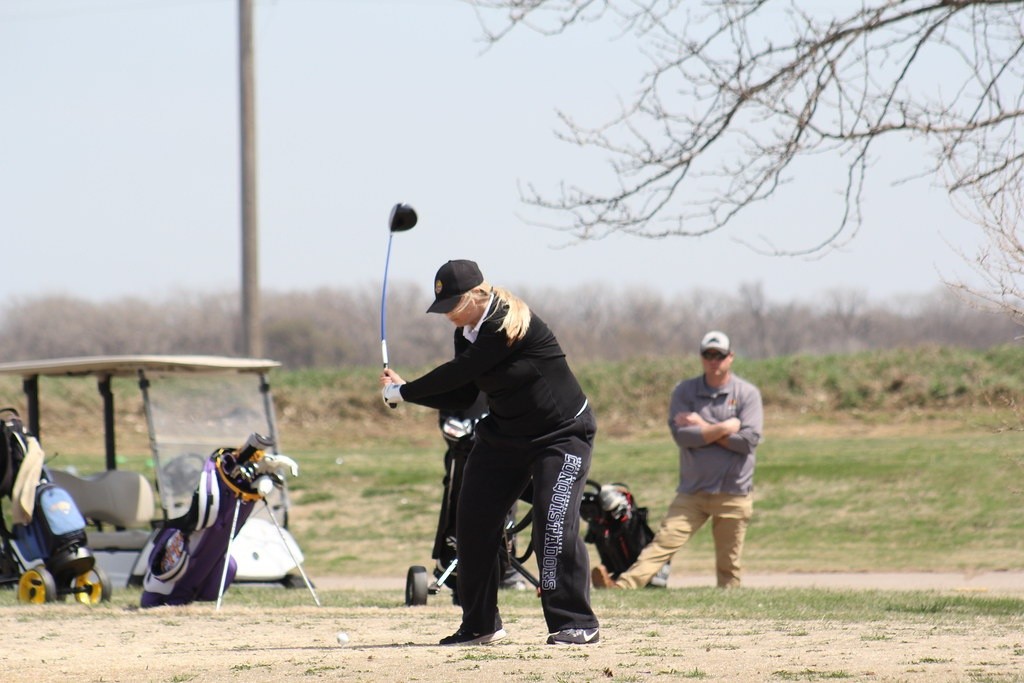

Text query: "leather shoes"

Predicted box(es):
[591, 565, 620, 589]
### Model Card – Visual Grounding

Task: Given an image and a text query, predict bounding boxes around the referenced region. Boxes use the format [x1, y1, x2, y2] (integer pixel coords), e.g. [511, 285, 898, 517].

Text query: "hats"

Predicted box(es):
[700, 331, 729, 355]
[426, 259, 483, 314]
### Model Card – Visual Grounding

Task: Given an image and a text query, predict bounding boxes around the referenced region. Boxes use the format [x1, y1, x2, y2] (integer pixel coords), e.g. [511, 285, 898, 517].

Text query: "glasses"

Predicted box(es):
[701, 352, 732, 360]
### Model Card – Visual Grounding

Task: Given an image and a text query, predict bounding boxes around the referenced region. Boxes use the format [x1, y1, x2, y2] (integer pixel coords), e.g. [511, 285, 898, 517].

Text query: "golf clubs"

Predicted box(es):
[379, 202, 418, 410]
[222, 432, 274, 498]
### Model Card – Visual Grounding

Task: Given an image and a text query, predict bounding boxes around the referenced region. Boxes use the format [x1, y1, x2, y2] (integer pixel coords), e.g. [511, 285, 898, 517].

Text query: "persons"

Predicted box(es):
[590, 331, 764, 590]
[381, 258, 600, 648]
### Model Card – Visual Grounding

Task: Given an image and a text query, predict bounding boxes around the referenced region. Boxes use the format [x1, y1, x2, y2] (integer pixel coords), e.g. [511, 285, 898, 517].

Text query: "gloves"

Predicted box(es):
[380, 384, 403, 411]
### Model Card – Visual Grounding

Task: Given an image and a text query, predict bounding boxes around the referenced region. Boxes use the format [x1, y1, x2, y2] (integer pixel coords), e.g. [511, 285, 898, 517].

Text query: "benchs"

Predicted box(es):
[51, 468, 153, 553]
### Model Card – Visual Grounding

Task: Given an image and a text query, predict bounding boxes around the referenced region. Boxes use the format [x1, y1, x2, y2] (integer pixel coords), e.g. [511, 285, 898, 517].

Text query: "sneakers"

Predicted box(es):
[440, 620, 505, 646]
[546, 625, 600, 646]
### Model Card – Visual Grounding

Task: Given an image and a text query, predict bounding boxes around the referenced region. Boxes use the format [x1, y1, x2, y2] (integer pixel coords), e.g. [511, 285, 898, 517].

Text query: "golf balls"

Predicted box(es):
[337, 634, 349, 645]
